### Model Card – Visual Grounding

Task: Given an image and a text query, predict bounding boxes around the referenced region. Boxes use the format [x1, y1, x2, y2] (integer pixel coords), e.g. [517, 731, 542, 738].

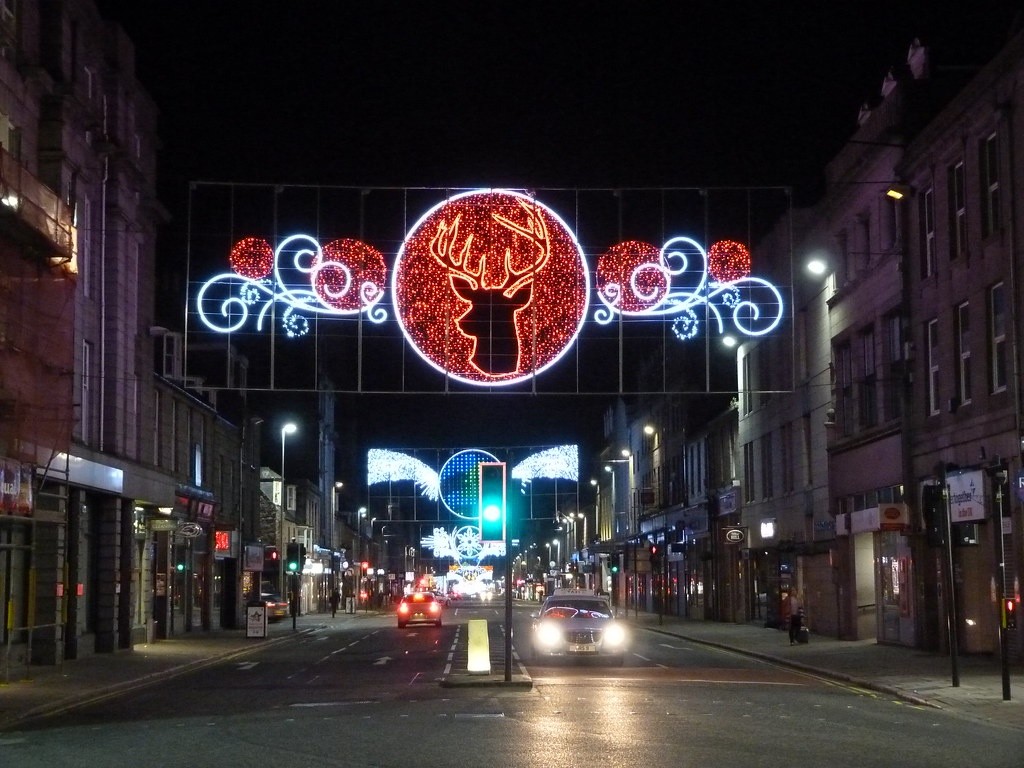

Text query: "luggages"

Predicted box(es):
[800, 617, 809, 643]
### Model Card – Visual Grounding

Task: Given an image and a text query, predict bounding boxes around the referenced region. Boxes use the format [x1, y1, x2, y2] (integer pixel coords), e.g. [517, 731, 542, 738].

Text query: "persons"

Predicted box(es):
[782, 587, 804, 643]
[329, 588, 341, 619]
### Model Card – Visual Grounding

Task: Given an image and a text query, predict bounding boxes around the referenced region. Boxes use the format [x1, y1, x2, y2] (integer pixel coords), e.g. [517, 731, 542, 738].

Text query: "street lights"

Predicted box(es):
[330, 481, 342, 557]
[357, 508, 368, 566]
[278, 423, 296, 598]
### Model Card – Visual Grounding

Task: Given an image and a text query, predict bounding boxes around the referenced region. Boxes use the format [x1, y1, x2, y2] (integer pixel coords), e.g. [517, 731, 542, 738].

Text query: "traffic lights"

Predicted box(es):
[361, 562, 369, 578]
[285, 543, 300, 572]
[480, 460, 506, 542]
[652, 545, 660, 555]
[607, 554, 612, 569]
[298, 541, 307, 571]
[611, 553, 619, 573]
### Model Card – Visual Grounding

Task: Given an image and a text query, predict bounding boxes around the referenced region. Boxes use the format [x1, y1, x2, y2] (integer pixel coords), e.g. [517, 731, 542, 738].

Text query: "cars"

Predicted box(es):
[396, 592, 442, 627]
[261, 593, 288, 621]
[596, 591, 610, 603]
[531, 588, 627, 666]
[430, 590, 452, 607]
